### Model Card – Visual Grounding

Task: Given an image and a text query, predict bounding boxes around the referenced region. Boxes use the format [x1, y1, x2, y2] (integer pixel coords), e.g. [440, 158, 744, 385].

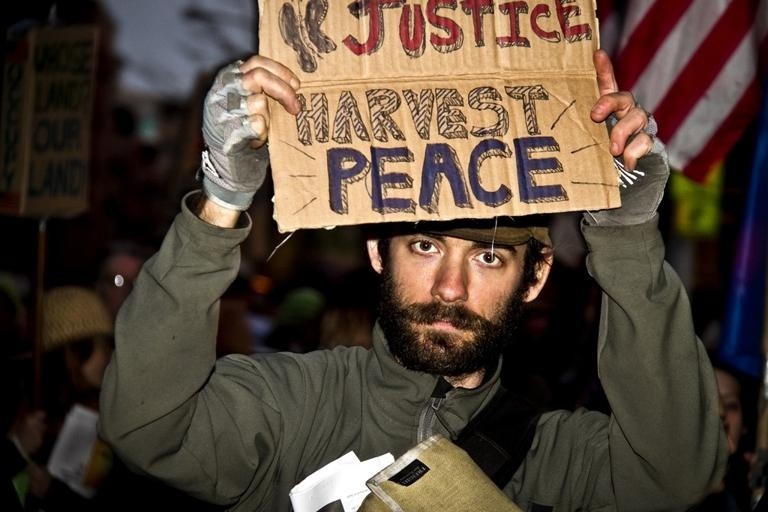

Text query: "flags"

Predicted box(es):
[594, 0, 763, 183]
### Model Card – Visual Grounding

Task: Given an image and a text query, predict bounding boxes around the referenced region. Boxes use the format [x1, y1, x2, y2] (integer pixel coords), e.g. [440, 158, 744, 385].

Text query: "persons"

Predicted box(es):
[1, 211, 767, 510]
[100, 53, 731, 512]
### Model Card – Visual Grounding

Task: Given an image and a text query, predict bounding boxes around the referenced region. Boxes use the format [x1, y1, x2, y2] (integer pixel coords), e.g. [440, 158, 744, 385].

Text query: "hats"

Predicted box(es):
[43, 287, 113, 348]
